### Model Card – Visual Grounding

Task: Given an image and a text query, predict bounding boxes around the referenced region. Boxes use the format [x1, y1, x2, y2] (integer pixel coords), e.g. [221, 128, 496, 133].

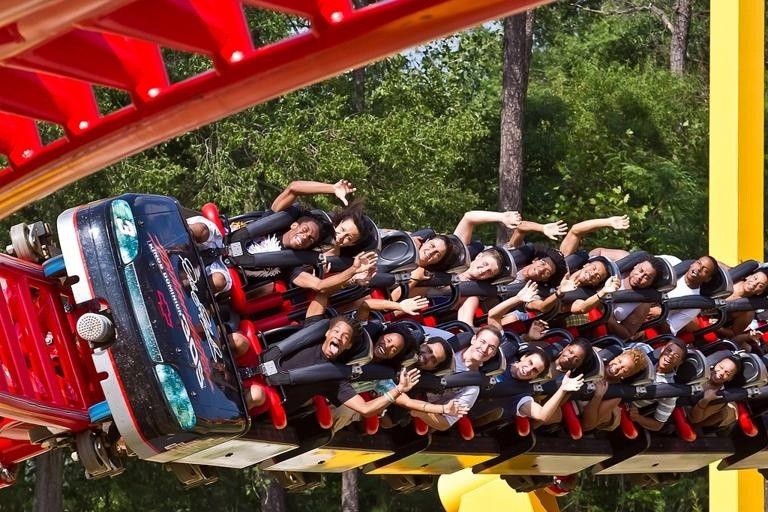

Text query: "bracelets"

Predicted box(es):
[394, 385, 402, 395]
[555, 290, 565, 299]
[383, 300, 389, 312]
[411, 278, 421, 282]
[341, 284, 346, 289]
[384, 392, 396, 403]
[443, 405, 444, 413]
[423, 403, 432, 412]
[597, 293, 603, 299]
[696, 402, 706, 409]
[348, 277, 356, 285]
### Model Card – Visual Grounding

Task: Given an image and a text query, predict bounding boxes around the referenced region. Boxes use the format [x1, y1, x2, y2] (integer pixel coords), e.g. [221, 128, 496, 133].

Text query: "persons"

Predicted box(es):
[185, 178, 768, 431]
